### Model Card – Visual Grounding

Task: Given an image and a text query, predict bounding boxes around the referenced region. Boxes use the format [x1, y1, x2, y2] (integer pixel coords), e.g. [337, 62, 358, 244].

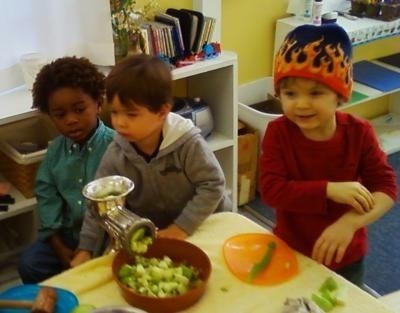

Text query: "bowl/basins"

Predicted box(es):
[110, 235, 212, 312]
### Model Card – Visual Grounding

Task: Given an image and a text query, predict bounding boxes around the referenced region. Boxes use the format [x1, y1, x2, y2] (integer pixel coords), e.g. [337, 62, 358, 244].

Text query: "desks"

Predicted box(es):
[37, 211, 378, 312]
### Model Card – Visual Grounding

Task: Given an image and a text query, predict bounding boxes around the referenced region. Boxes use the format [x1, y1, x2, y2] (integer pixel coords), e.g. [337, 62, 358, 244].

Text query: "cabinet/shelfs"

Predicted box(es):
[0, 51, 238, 292]
[272, 17, 400, 158]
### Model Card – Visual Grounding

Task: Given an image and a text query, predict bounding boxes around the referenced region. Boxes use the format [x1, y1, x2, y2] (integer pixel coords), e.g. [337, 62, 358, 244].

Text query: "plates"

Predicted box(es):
[1, 282, 79, 313]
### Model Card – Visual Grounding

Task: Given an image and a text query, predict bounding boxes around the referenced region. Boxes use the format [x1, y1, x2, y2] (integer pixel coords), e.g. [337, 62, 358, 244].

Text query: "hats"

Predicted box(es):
[273, 23, 353, 102]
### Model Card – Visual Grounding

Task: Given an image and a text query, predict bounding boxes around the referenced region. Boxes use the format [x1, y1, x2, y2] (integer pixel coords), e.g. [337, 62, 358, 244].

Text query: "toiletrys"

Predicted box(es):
[323, 10, 340, 24]
[305, 0, 311, 22]
[312, 0, 322, 27]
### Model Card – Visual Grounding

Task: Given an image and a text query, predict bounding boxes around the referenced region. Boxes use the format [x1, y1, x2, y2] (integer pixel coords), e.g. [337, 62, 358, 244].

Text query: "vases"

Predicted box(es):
[126, 34, 142, 56]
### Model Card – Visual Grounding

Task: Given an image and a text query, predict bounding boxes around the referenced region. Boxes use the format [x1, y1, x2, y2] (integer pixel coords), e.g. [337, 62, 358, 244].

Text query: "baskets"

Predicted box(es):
[0, 139, 48, 199]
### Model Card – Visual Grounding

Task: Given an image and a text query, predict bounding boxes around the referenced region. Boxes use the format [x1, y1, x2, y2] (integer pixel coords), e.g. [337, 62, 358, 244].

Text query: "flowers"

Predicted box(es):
[111, 0, 151, 56]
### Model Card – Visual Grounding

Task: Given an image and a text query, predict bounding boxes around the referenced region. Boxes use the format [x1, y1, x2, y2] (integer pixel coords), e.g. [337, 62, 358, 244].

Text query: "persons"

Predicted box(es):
[71, 54, 225, 266]
[258, 24, 398, 285]
[18, 56, 119, 282]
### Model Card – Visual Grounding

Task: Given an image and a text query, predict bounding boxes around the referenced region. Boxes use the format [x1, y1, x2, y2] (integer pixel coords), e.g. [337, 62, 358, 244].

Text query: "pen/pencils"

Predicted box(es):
[337, 11, 356, 20]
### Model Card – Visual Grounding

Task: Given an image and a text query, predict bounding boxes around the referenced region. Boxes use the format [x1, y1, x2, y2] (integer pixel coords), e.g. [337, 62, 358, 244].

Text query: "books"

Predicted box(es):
[128, 8, 216, 66]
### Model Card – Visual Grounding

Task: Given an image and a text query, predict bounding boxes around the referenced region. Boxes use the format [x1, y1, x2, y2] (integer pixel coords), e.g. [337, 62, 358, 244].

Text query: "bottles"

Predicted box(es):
[312, 0, 324, 26]
